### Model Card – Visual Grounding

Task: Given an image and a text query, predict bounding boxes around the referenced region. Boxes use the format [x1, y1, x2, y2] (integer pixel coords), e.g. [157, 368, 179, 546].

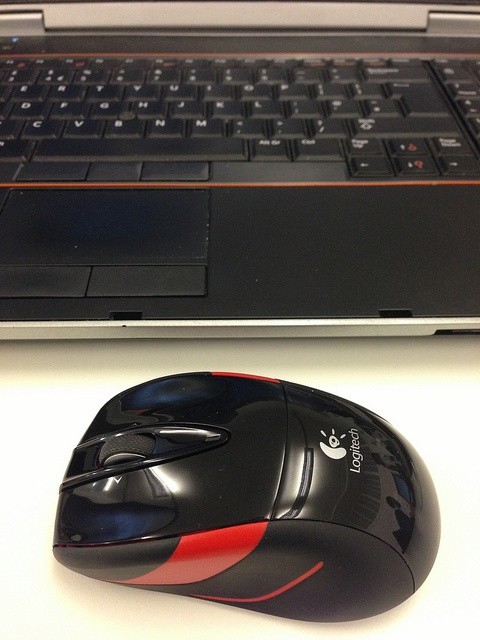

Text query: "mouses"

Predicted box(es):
[52, 369, 442, 625]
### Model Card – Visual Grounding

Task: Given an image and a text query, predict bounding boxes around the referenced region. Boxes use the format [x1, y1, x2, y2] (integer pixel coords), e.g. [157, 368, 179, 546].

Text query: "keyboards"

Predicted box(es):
[1, 3, 480, 342]
